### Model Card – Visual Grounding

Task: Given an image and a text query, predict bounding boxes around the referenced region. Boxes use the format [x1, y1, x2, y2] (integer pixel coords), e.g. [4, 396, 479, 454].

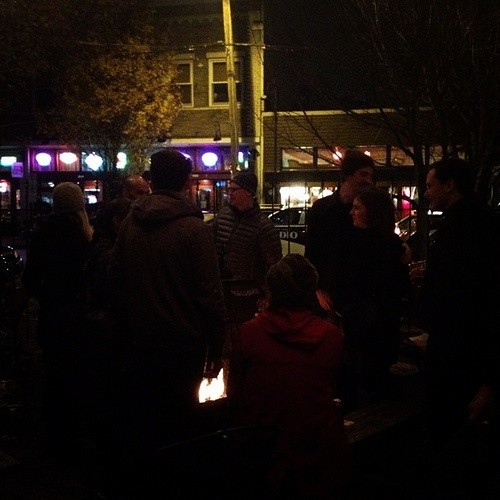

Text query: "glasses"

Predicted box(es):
[227, 187, 244, 194]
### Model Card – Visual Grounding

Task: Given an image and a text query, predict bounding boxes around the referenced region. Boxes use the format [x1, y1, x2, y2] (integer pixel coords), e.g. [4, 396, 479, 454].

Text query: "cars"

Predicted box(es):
[392, 215, 415, 238]
[0, 245, 25, 286]
[255, 202, 313, 258]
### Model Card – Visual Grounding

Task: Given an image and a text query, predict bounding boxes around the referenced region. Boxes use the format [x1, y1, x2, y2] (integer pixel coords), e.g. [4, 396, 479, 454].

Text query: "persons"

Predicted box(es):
[307, 157, 416, 487]
[408, 161, 500, 448]
[0, 149, 228, 500]
[205, 173, 282, 355]
[223, 254, 342, 500]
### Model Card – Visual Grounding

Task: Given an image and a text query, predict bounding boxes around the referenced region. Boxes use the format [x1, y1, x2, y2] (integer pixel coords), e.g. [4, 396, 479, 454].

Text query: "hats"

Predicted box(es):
[142, 150, 193, 189]
[53, 182, 87, 211]
[230, 173, 258, 196]
[275, 254, 319, 298]
